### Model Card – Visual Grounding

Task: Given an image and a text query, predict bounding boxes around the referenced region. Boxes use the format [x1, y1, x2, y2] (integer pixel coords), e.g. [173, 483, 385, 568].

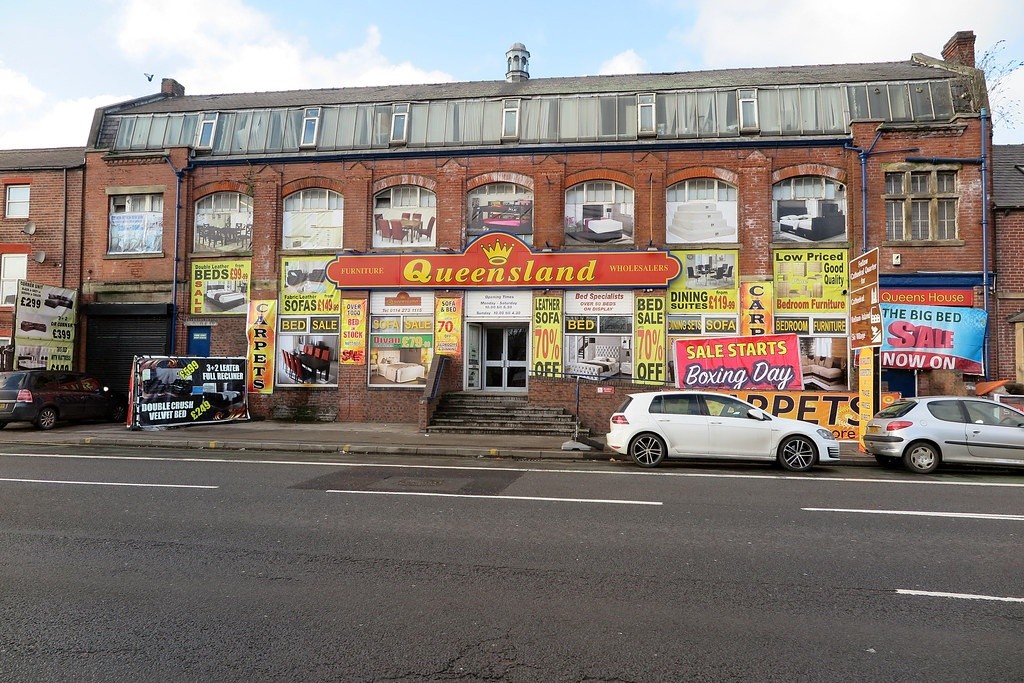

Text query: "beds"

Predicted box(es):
[206, 289, 245, 312]
[581, 217, 624, 241]
[378, 360, 425, 383]
[779, 214, 846, 241]
[571, 358, 619, 381]
[202, 390, 242, 408]
[480, 216, 532, 234]
[18, 361, 48, 370]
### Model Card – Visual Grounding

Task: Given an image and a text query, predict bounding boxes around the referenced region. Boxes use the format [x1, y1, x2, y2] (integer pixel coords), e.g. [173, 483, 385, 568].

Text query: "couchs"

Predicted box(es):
[800, 355, 847, 391]
[287, 269, 308, 286]
[21, 320, 46, 333]
[143, 379, 166, 395]
[44, 294, 73, 310]
[308, 268, 327, 283]
[170, 379, 193, 395]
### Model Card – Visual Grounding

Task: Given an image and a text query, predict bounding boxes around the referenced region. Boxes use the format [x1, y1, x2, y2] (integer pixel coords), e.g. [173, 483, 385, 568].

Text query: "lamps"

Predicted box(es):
[625, 351, 630, 363]
[606, 208, 612, 219]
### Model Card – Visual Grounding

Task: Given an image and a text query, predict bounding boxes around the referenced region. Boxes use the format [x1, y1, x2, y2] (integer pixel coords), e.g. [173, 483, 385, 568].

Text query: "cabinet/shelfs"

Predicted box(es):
[777, 261, 824, 298]
[285, 211, 343, 248]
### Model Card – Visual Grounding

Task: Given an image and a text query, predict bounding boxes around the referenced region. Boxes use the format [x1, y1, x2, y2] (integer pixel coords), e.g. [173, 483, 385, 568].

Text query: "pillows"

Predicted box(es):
[600, 358, 609, 362]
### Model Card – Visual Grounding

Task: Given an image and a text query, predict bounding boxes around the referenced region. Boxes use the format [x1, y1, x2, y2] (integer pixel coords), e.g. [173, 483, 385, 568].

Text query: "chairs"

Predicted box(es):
[417, 217, 436, 242]
[284, 351, 292, 378]
[295, 357, 312, 384]
[280, 349, 288, 369]
[412, 213, 423, 237]
[320, 350, 329, 380]
[374, 214, 385, 234]
[378, 219, 393, 243]
[307, 344, 314, 357]
[390, 220, 408, 245]
[197, 219, 252, 249]
[289, 353, 298, 381]
[400, 212, 410, 233]
[687, 263, 734, 287]
[304, 345, 308, 354]
[314, 347, 322, 358]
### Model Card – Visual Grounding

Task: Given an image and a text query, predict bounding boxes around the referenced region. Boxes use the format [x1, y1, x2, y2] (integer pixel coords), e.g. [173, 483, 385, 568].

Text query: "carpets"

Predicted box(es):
[566, 230, 632, 245]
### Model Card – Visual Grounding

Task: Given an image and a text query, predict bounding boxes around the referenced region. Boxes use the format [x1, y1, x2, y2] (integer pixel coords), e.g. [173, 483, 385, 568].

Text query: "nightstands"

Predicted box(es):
[621, 361, 632, 375]
[370, 364, 378, 378]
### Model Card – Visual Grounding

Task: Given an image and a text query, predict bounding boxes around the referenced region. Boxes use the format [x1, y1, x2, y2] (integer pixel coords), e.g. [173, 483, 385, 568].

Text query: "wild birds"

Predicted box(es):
[144, 73, 154, 82]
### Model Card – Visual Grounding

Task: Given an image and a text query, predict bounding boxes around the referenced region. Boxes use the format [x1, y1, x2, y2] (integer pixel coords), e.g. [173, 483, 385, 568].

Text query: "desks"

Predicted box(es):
[386, 218, 423, 243]
[296, 352, 330, 384]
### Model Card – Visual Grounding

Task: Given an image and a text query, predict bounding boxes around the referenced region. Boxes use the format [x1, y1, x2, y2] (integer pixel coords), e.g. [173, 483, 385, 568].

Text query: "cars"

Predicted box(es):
[863, 394, 1024, 475]
[0, 367, 110, 432]
[607, 389, 841, 474]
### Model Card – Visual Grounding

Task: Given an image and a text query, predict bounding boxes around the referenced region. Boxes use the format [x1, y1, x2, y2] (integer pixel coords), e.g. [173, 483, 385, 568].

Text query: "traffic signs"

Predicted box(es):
[847, 245, 886, 355]
